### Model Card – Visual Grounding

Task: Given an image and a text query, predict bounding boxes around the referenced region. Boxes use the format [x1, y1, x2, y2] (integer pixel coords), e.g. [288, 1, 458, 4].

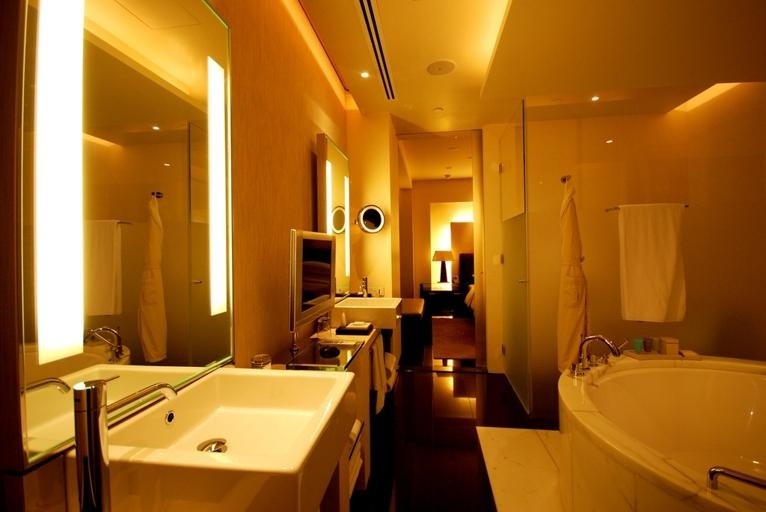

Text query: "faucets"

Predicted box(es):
[360, 276, 369, 298]
[568, 334, 623, 381]
[19, 377, 72, 395]
[73, 375, 177, 451]
[89, 327, 127, 364]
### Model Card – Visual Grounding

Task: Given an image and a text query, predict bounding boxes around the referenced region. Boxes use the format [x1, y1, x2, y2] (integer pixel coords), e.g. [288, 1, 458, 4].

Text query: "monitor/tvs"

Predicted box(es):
[289, 227, 336, 332]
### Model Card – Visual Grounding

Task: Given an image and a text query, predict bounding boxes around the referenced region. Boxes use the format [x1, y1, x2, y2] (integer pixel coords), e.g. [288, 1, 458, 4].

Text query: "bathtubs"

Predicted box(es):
[557, 349, 766, 511]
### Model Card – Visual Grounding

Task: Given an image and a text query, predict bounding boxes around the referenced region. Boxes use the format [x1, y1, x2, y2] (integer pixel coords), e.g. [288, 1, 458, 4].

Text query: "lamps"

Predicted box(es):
[433, 251, 455, 282]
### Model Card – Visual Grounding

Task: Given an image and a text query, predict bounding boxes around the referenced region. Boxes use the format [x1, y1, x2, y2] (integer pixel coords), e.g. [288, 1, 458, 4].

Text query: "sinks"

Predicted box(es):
[19, 364, 207, 463]
[108, 367, 357, 474]
[334, 297, 402, 328]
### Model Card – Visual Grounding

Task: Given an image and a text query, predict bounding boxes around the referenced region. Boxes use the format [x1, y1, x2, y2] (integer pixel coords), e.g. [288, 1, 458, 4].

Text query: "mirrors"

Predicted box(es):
[317, 132, 351, 296]
[21, 0, 229, 468]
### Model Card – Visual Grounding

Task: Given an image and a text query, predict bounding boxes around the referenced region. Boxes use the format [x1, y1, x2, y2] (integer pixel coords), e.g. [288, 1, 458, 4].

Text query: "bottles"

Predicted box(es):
[247, 353, 271, 369]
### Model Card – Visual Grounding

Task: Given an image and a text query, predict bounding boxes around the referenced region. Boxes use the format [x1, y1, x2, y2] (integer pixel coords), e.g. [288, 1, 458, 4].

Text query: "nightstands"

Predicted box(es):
[431, 282, 453, 316]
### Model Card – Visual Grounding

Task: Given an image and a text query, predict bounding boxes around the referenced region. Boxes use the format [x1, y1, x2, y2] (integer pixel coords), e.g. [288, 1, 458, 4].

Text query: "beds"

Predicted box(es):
[460, 253, 474, 317]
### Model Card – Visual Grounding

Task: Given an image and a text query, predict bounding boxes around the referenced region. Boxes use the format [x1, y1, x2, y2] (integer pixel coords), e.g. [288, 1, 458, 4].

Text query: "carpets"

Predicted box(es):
[432, 315, 477, 359]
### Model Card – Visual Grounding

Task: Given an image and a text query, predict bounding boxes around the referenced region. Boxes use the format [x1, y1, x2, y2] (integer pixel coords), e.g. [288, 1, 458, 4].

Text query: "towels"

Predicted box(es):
[385, 352, 398, 392]
[371, 334, 387, 415]
[83, 219, 122, 318]
[619, 202, 687, 322]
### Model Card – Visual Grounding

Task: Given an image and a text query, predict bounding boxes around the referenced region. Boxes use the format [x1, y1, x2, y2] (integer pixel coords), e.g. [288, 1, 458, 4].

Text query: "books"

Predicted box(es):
[335, 323, 374, 336]
[345, 320, 371, 330]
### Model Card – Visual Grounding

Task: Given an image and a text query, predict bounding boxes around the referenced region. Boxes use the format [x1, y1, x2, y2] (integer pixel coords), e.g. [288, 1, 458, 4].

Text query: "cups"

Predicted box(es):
[633, 336, 659, 354]
[317, 317, 332, 339]
